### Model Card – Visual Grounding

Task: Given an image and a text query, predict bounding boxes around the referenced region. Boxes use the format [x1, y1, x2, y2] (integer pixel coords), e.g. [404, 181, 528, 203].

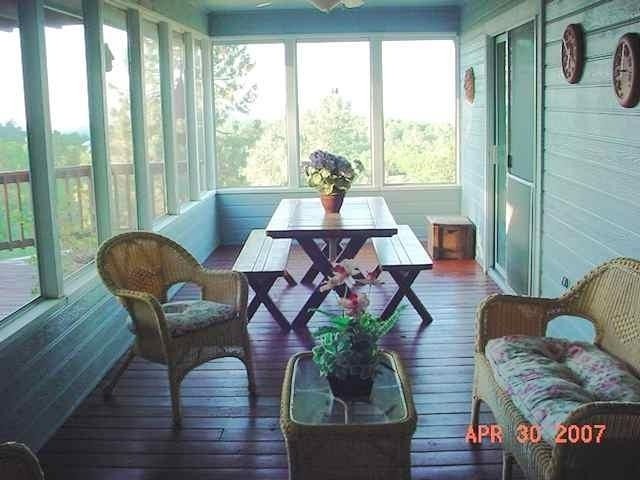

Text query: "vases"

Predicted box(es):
[315, 186, 345, 213]
[322, 360, 374, 399]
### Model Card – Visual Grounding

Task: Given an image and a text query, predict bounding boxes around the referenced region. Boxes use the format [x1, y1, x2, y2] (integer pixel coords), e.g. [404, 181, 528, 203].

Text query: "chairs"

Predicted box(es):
[96, 231, 259, 429]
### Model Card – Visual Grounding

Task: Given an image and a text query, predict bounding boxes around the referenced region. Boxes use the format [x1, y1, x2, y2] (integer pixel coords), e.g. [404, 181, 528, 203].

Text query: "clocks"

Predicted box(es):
[560, 24, 582, 85]
[612, 33, 639, 108]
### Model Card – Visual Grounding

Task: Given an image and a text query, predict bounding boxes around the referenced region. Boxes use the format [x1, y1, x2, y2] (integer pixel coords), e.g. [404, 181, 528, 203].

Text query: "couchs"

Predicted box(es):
[472, 258, 640, 480]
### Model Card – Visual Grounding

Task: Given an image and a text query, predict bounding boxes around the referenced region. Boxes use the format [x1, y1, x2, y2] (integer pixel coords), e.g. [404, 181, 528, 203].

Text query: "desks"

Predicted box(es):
[266, 196, 398, 331]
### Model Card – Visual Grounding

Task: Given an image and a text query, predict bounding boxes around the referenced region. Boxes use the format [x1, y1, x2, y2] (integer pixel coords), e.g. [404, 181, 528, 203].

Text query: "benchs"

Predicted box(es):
[231, 226, 296, 333]
[369, 224, 435, 324]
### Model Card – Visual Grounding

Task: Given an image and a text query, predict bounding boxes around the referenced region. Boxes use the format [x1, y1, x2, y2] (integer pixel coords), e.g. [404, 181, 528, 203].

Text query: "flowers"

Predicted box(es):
[298, 261, 403, 375]
[304, 148, 362, 192]
